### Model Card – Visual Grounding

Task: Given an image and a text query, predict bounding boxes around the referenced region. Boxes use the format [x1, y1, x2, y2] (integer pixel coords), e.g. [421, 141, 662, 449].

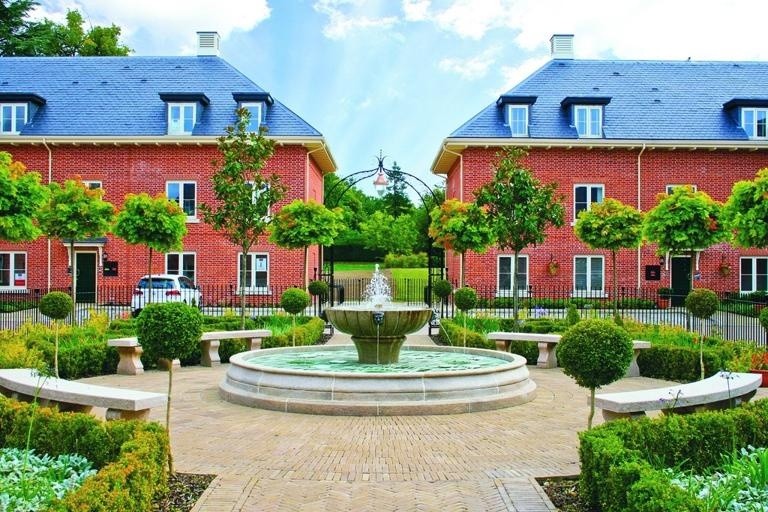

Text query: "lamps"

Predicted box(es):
[658, 255, 666, 264]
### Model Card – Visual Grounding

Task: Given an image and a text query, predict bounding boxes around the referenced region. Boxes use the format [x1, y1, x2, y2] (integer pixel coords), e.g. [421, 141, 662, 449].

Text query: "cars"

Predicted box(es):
[131, 274, 202, 320]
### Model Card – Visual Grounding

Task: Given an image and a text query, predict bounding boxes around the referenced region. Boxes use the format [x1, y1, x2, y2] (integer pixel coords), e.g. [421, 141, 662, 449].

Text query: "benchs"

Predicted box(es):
[110, 327, 272, 375]
[0, 363, 154, 424]
[586, 372, 764, 420]
[482, 333, 650, 381]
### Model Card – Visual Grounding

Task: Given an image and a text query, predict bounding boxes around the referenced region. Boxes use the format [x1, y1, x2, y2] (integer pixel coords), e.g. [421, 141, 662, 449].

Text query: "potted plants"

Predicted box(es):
[750, 308, 768, 385]
[655, 286, 674, 309]
